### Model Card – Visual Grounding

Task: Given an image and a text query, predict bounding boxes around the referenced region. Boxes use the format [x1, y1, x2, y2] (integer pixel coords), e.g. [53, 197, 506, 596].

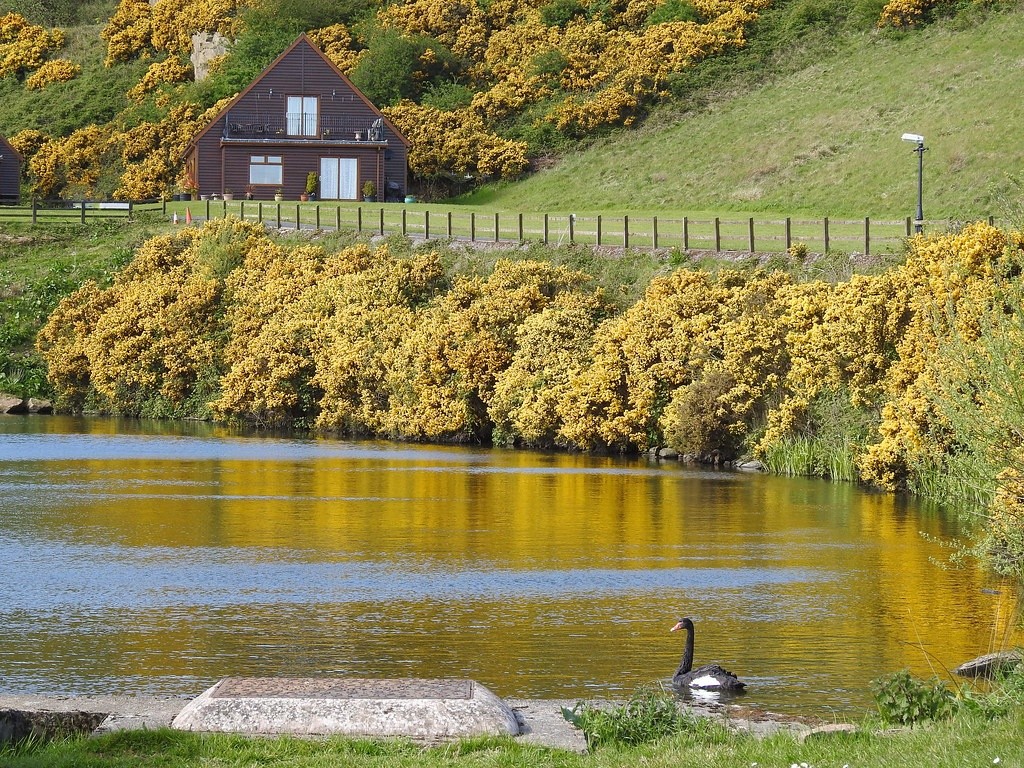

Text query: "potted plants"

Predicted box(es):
[178, 172, 202, 201]
[361, 181, 377, 202]
[170, 184, 180, 201]
[273, 187, 285, 201]
[404, 195, 417, 203]
[244, 184, 256, 200]
[300, 170, 321, 201]
[221, 188, 236, 200]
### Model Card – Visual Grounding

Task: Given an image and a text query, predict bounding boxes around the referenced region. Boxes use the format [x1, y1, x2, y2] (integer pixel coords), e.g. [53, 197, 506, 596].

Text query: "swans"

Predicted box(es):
[669, 617, 747, 692]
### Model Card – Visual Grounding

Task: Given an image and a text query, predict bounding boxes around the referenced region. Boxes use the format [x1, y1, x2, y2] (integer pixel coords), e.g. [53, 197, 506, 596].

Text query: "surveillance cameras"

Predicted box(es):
[901, 133, 924, 144]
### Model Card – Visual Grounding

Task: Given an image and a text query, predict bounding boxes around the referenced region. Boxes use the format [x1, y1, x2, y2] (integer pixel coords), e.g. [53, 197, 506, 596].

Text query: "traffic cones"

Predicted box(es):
[173, 210, 179, 225]
[186, 206, 193, 225]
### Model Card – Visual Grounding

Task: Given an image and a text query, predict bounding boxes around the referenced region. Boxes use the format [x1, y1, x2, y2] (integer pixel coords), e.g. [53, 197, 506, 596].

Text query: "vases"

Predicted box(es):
[200, 195, 211, 200]
[211, 192, 220, 200]
